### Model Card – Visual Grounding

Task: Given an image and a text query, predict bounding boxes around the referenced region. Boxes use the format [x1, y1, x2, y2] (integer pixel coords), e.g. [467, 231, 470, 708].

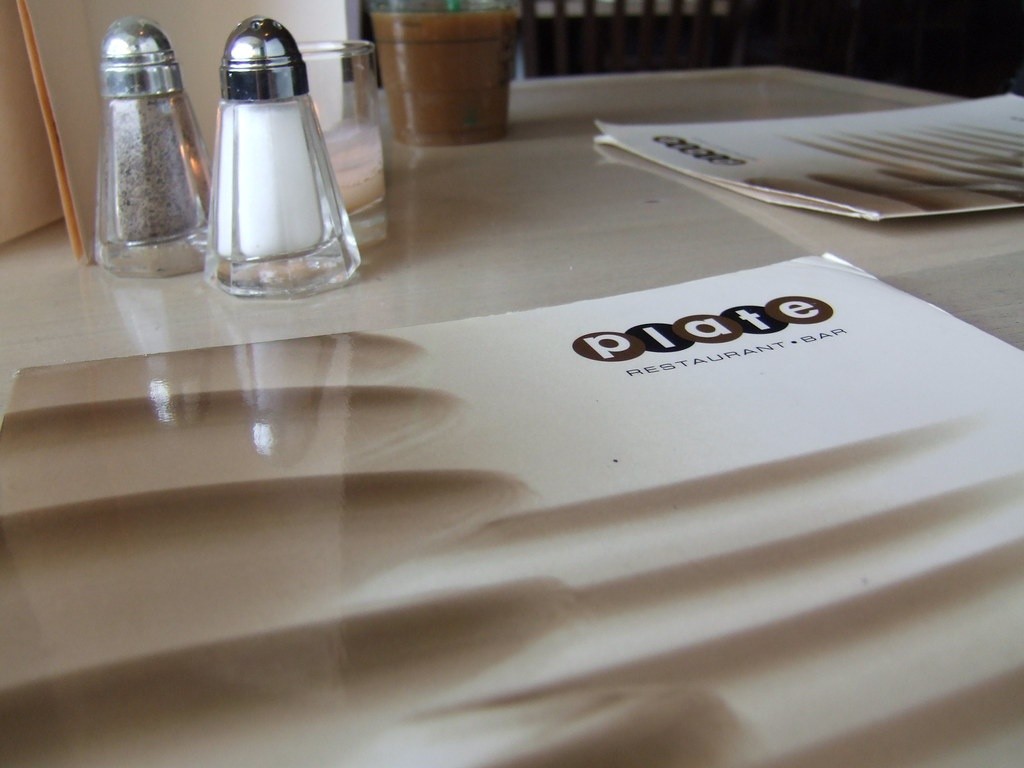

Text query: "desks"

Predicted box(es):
[0, 65, 1024, 768]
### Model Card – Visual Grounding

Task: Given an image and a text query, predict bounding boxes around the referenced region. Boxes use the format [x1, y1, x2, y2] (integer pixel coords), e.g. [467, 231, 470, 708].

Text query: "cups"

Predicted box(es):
[367, 2, 522, 143]
[301, 43, 390, 252]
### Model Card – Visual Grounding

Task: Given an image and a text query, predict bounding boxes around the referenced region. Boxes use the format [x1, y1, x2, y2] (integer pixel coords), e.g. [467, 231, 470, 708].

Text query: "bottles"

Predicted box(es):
[210, 15, 360, 296]
[95, 16, 209, 278]
